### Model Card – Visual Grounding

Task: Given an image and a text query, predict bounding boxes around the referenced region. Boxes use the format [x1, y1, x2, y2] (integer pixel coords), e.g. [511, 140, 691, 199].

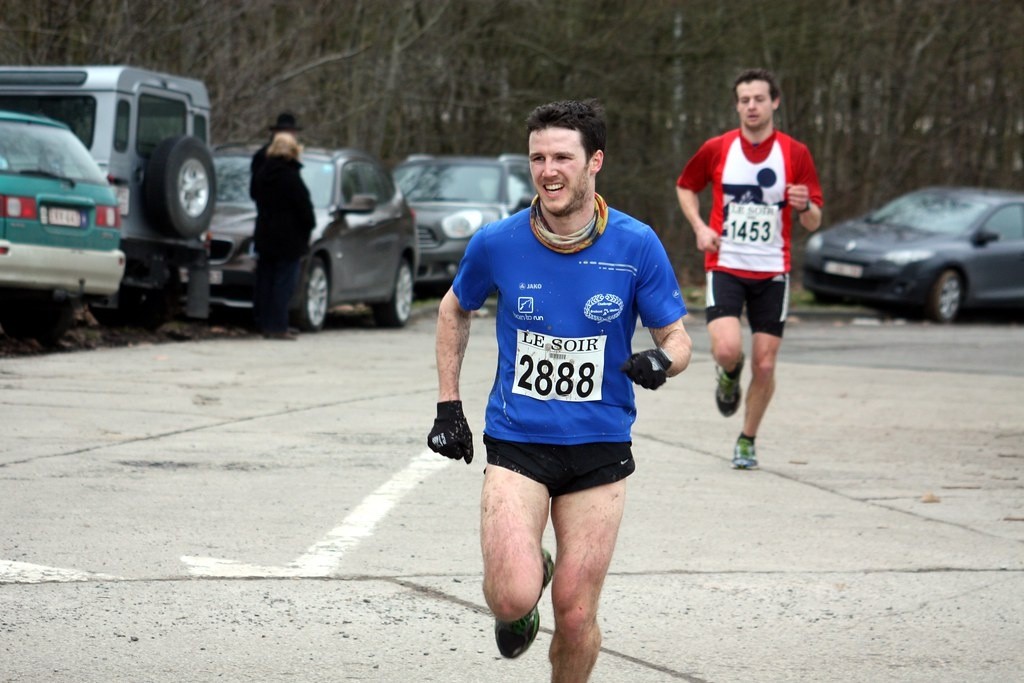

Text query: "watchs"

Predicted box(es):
[655, 347, 673, 370]
[797, 199, 811, 213]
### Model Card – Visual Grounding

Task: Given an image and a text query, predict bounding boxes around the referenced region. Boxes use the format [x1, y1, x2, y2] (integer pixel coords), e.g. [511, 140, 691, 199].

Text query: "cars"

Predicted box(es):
[178, 142, 420, 334]
[798, 187, 1023, 325]
[0, 109, 124, 342]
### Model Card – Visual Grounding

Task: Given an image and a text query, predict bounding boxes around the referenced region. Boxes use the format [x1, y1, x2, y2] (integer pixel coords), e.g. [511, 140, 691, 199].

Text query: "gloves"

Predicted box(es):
[427, 400, 474, 465]
[619, 348, 672, 390]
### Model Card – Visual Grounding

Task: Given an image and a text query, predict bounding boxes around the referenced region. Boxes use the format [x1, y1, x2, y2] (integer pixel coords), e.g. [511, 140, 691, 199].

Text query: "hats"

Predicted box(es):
[268, 112, 304, 131]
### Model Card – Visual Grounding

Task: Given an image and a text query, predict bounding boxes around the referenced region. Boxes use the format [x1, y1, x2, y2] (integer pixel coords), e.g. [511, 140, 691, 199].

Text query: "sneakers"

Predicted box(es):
[495, 548, 554, 659]
[715, 361, 744, 416]
[732, 437, 759, 470]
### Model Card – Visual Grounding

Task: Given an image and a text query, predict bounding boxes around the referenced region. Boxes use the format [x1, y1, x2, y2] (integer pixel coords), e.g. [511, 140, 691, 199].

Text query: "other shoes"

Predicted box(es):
[264, 330, 295, 339]
[287, 327, 300, 333]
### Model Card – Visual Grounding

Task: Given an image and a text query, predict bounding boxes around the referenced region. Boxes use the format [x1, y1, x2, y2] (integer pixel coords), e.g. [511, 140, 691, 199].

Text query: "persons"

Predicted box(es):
[429, 97, 693, 683]
[675, 67, 825, 471]
[248, 113, 316, 341]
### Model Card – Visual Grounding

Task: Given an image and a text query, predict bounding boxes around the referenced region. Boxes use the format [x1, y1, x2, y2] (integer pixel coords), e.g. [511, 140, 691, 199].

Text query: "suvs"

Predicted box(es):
[387, 152, 536, 283]
[0, 63, 218, 333]
